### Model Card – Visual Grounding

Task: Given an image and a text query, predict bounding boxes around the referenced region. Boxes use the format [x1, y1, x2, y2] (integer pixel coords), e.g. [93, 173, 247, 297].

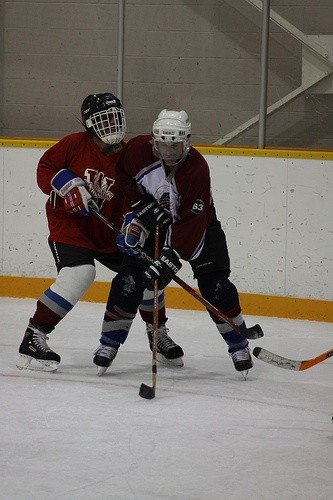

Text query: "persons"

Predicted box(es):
[15, 93, 185, 373]
[122, 107, 253, 382]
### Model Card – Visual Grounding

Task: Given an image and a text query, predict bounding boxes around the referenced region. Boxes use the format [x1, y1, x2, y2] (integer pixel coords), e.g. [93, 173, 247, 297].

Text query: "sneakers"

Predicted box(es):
[144, 322, 184, 367]
[16, 328, 61, 372]
[231, 346, 253, 382]
[93, 342, 117, 375]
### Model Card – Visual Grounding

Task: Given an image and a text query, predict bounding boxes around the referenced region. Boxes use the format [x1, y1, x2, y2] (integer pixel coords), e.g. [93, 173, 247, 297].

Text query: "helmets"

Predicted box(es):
[152, 109, 191, 144]
[81, 93, 127, 147]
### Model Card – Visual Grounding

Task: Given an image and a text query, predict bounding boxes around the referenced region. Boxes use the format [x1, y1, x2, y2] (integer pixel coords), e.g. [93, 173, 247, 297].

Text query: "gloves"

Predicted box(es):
[144, 252, 182, 292]
[49, 170, 92, 216]
[115, 212, 150, 256]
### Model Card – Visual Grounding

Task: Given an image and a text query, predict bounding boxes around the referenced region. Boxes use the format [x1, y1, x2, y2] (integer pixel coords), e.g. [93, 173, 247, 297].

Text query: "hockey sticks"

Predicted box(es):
[253, 345, 332, 372]
[87, 203, 266, 340]
[138, 222, 160, 400]
